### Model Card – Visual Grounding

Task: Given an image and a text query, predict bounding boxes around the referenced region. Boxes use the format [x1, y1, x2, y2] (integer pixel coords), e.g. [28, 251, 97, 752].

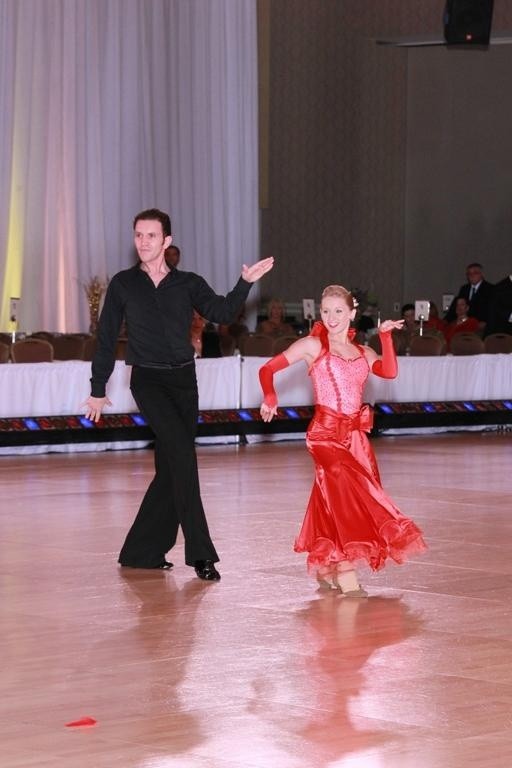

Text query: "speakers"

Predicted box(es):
[443, 0, 493, 51]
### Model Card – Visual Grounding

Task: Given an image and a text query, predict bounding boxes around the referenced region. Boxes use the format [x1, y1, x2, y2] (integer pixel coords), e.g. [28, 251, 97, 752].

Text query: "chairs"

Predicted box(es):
[2, 313, 511, 363]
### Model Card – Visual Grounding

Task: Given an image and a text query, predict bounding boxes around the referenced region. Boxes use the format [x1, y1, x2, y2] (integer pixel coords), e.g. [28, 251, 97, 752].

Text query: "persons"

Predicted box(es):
[80, 206, 275, 580]
[207, 303, 250, 347]
[458, 261, 501, 325]
[190, 307, 224, 360]
[163, 245, 180, 267]
[350, 288, 444, 356]
[481, 271, 512, 342]
[257, 284, 431, 597]
[441, 293, 483, 353]
[253, 297, 296, 337]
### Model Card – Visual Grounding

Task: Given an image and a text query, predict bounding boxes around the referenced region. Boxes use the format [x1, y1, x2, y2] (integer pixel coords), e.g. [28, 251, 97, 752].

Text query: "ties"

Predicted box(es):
[470, 288, 476, 301]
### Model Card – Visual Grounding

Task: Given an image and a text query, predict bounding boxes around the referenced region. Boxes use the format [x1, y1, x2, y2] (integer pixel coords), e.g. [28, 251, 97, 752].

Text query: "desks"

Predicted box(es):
[0, 354, 242, 457]
[240, 352, 512, 445]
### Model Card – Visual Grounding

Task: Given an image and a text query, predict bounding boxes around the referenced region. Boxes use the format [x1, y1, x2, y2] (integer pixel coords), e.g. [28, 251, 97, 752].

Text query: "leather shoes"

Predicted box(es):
[194, 559, 222, 582]
[315, 567, 340, 594]
[331, 565, 370, 600]
[118, 556, 174, 570]
